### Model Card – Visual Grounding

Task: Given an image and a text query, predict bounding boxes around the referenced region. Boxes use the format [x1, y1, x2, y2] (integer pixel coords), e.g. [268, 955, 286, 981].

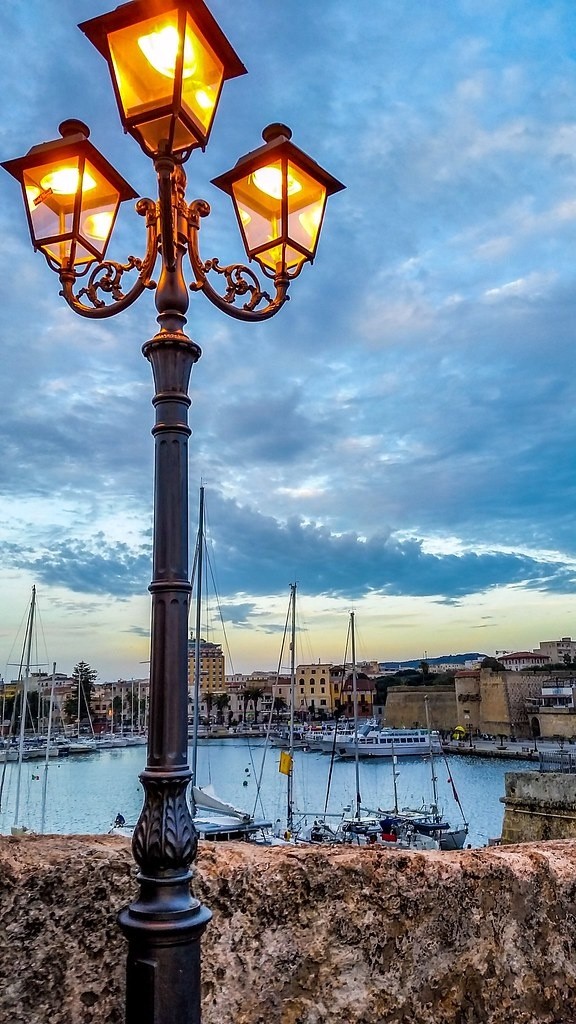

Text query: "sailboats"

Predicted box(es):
[0, 662, 148, 761]
[188, 477, 470, 851]
[0, 583, 137, 838]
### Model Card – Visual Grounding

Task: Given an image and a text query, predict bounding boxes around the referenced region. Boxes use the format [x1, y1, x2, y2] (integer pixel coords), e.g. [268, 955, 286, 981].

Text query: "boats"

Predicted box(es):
[188, 715, 359, 752]
[333, 718, 444, 756]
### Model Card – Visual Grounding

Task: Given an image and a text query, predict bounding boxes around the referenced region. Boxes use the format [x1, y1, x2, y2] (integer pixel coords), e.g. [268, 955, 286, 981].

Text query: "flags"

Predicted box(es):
[279, 752, 292, 776]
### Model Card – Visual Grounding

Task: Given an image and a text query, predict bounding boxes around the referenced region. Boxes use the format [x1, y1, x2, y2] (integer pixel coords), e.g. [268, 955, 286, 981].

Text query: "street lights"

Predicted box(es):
[0, 0, 347, 1024]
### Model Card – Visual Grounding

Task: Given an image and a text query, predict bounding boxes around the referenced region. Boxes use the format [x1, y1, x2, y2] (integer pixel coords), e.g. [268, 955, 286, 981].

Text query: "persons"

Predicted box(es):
[116, 813, 125, 827]
[440, 727, 454, 742]
